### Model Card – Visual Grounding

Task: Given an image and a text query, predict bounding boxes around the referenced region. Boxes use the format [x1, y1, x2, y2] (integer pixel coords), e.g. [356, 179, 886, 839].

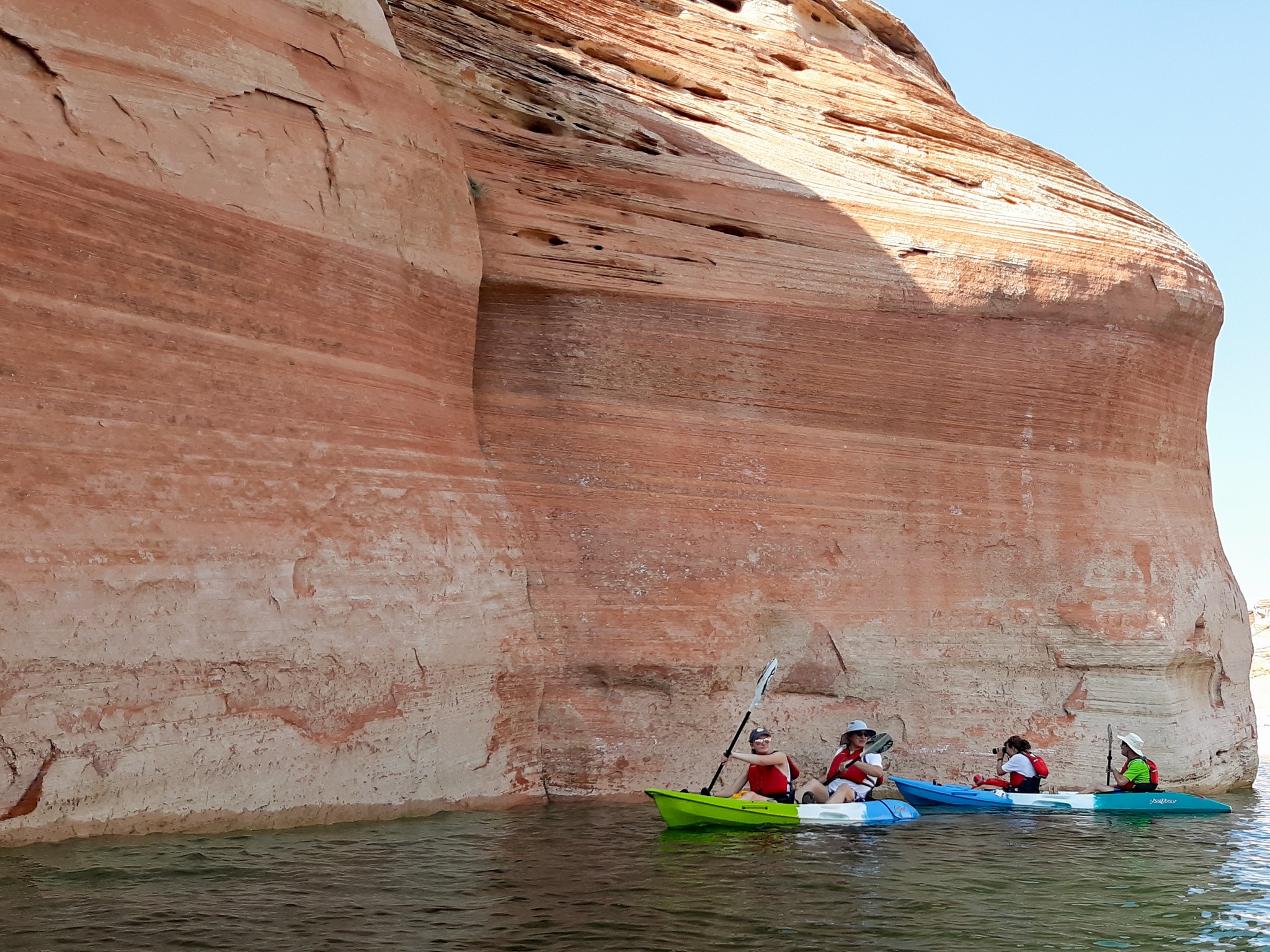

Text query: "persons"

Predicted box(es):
[933, 735, 1041, 794]
[1075, 732, 1151, 794]
[680, 726, 794, 804]
[794, 721, 883, 804]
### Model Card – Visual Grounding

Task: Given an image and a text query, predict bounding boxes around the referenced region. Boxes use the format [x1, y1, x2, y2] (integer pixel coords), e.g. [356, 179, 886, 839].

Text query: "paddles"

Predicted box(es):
[1106, 723, 1113, 785]
[821, 731, 893, 787]
[706, 655, 779, 793]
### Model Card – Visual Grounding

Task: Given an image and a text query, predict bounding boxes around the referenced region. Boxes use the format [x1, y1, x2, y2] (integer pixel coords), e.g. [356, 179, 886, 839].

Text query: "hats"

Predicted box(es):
[839, 720, 877, 746]
[1117, 733, 1144, 756]
[748, 728, 771, 742]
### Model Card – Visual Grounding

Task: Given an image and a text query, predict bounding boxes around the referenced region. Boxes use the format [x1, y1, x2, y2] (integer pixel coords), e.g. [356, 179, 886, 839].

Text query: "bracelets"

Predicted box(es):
[997, 758, 1002, 762]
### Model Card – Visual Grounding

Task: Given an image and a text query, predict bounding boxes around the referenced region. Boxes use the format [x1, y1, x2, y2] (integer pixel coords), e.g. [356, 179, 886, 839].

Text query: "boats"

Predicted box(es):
[887, 773, 1233, 814]
[641, 786, 922, 832]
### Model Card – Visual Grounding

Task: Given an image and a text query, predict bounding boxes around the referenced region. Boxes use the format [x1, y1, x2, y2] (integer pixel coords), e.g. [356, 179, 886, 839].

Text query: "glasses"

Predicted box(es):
[752, 736, 771, 744]
[1122, 741, 1126, 746]
[853, 731, 869, 737]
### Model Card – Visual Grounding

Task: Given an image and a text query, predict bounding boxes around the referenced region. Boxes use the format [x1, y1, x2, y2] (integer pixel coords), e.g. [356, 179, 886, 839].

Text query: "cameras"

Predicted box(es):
[992, 746, 1007, 754]
